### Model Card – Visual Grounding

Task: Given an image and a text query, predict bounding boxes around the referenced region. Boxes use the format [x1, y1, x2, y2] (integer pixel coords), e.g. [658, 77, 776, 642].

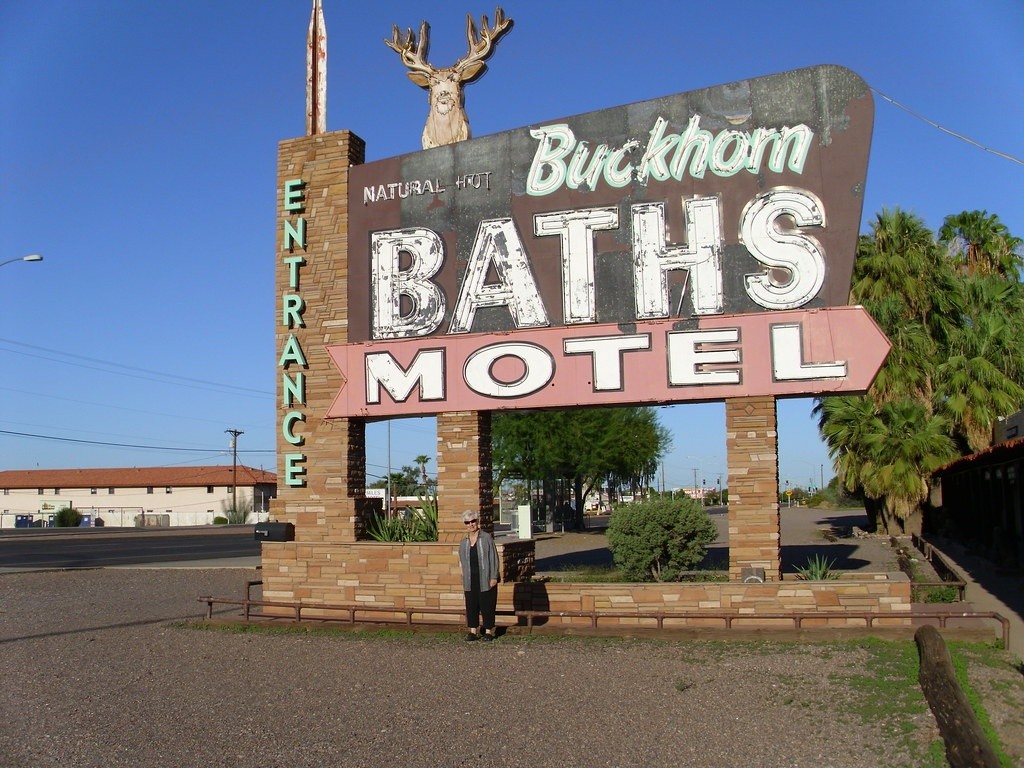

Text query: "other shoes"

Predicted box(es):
[464, 633, 478, 641]
[482, 634, 495, 641]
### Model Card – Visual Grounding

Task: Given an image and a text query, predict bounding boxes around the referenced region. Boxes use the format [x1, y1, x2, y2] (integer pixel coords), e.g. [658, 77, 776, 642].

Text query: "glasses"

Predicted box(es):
[464, 519, 476, 524]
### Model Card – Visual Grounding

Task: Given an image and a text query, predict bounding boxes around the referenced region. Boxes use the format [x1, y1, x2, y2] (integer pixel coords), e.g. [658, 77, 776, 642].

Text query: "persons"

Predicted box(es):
[458, 509, 501, 641]
